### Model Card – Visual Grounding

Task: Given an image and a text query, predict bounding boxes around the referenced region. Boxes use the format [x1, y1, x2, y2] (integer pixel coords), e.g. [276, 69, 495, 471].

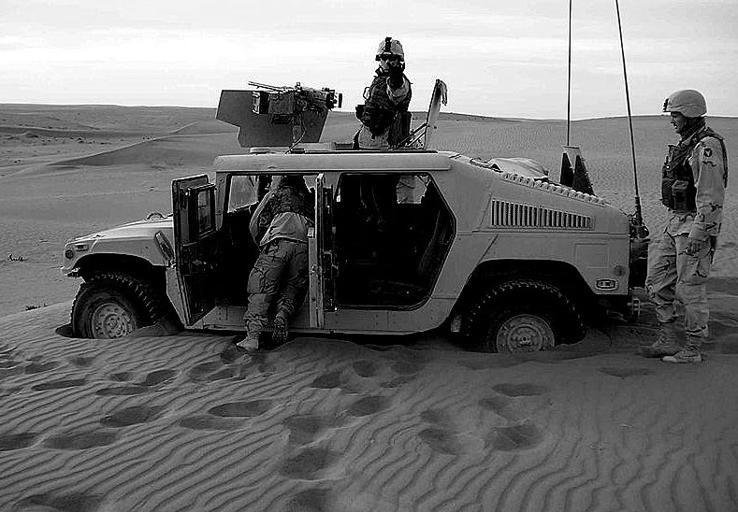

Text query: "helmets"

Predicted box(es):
[661, 90, 707, 118]
[375, 37, 405, 62]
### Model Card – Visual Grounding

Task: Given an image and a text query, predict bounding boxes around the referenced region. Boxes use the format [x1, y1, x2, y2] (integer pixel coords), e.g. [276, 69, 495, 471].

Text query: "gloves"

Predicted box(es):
[387, 62, 406, 89]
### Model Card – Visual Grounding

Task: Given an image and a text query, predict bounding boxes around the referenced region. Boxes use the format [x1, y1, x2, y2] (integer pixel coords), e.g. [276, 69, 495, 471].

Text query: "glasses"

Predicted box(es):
[380, 55, 397, 62]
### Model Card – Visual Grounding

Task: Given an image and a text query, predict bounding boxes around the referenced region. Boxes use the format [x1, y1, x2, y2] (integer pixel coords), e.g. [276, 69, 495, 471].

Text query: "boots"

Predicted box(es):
[234, 336, 260, 351]
[272, 312, 289, 343]
[642, 325, 679, 357]
[662, 335, 703, 363]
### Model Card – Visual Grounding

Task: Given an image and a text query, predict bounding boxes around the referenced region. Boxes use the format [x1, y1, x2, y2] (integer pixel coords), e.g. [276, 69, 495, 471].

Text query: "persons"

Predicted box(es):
[235, 177, 315, 353]
[352, 35, 413, 229]
[635, 89, 729, 364]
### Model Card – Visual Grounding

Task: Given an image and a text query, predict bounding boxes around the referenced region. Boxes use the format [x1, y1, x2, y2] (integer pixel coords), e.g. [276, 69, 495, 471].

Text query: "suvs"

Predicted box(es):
[60, 81, 650, 354]
